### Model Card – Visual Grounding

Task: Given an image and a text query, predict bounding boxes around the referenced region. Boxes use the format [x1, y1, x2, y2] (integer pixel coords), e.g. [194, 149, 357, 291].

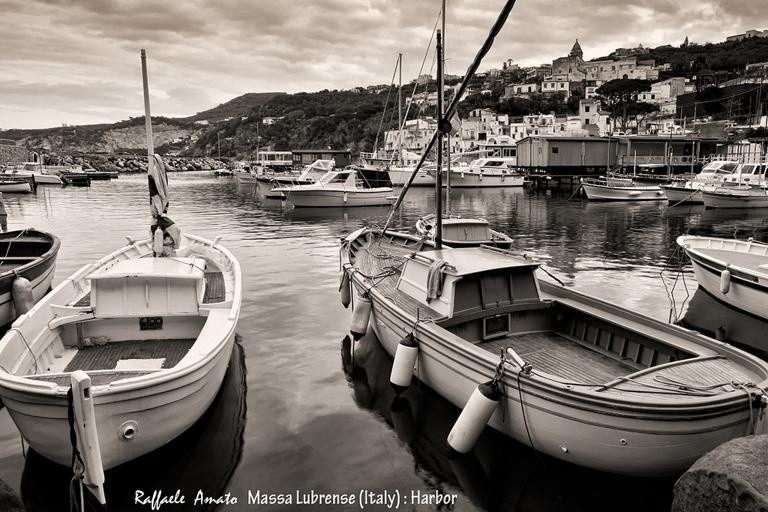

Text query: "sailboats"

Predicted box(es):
[360, 0, 445, 186]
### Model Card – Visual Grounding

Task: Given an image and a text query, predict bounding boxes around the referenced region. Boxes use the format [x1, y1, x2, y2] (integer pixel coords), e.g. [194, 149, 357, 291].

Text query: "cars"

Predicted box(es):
[656, 125, 691, 134]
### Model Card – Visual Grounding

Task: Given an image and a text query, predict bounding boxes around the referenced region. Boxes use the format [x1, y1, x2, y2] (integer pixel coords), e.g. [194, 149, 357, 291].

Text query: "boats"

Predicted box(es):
[1, 227, 61, 326]
[253, 160, 395, 208]
[332, 226, 761, 469]
[0, 163, 63, 193]
[426, 159, 522, 187]
[579, 161, 768, 208]
[672, 285, 768, 362]
[20, 332, 247, 512]
[660, 221, 766, 322]
[0, 230, 243, 505]
[346, 344, 672, 509]
